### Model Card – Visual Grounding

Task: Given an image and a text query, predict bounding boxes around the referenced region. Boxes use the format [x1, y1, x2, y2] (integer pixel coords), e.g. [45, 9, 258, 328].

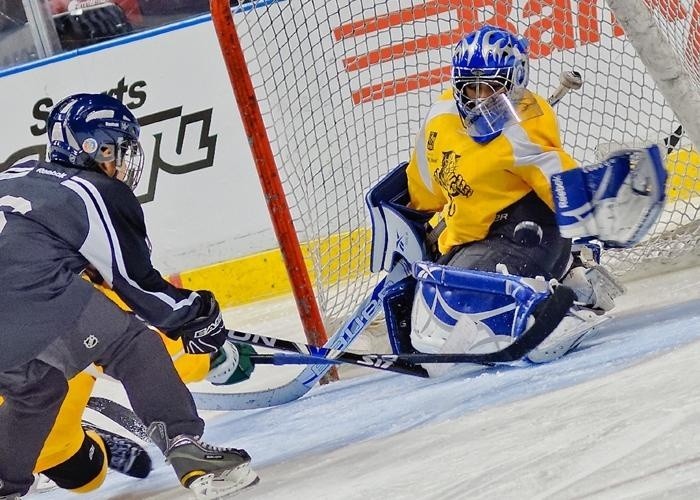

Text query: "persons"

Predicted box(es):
[27, 277, 254, 495]
[0, 90, 261, 499]
[363, 27, 666, 362]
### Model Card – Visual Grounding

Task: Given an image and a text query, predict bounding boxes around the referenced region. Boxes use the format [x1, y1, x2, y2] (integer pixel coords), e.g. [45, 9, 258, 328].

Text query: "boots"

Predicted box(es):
[147, 421, 251, 487]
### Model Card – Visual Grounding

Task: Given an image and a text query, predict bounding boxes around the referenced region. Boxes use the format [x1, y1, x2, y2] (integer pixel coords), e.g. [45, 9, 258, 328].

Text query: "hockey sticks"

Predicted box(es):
[251, 286, 576, 364]
[85, 396, 155, 444]
[190, 71, 581, 409]
[225, 314, 479, 379]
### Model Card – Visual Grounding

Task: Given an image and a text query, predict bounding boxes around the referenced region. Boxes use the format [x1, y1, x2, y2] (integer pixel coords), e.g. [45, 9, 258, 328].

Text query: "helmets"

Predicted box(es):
[46, 92, 145, 192]
[451, 25, 530, 115]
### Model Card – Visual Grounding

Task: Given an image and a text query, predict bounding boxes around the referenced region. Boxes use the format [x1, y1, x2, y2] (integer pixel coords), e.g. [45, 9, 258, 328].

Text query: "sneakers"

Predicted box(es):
[79, 419, 151, 478]
[522, 266, 614, 366]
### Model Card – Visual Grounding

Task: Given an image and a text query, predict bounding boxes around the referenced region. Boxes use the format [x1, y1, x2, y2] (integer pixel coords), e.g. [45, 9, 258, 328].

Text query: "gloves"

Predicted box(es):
[207, 339, 258, 385]
